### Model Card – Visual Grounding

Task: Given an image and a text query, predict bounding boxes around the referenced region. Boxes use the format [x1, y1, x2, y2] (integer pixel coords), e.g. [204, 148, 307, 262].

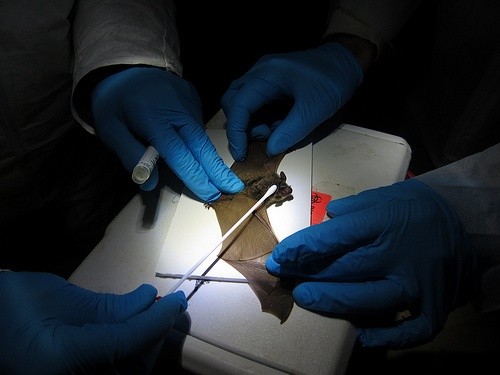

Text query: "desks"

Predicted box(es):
[65, 107, 412, 375]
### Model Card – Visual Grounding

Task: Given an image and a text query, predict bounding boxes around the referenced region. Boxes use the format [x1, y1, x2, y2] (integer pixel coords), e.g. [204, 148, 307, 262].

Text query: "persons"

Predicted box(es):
[218, 0, 499, 349]
[0, 0, 247, 374]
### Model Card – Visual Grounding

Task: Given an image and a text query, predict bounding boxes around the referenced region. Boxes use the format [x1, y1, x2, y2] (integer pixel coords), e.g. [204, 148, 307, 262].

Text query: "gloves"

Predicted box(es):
[91, 65, 244, 203]
[264, 178, 474, 350]
[222, 43, 363, 162]
[0, 271, 187, 375]
